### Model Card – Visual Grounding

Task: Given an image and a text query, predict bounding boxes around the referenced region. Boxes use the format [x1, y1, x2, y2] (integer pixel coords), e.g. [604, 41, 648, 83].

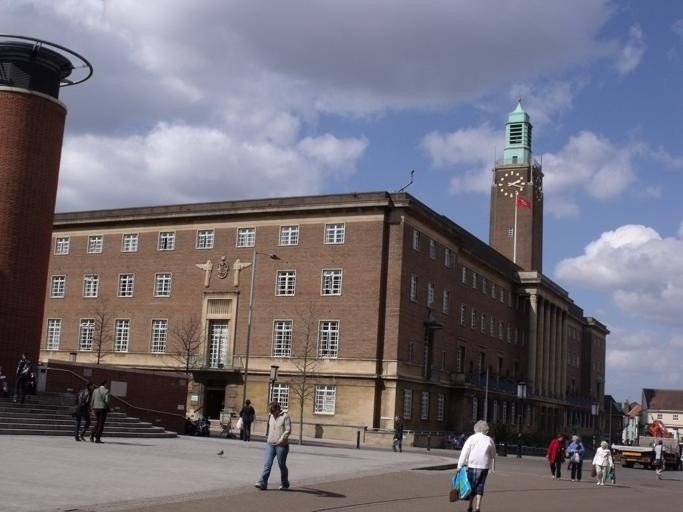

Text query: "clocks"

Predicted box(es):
[535, 175, 544, 202]
[498, 170, 525, 197]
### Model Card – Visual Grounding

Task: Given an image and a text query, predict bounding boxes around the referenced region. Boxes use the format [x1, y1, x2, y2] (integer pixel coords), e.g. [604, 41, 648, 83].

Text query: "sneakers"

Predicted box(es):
[253, 479, 289, 491]
[74, 434, 104, 443]
[550, 477, 608, 486]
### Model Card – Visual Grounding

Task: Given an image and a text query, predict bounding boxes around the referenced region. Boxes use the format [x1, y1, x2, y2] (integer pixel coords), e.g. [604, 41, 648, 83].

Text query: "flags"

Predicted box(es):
[516, 195, 531, 208]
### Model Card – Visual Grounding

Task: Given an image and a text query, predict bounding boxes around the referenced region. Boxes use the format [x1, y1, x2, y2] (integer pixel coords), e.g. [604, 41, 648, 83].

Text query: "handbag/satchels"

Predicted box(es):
[71, 405, 86, 419]
[607, 466, 616, 481]
[236, 417, 244, 430]
[651, 458, 662, 467]
[450, 467, 472, 500]
[590, 466, 597, 478]
[575, 452, 581, 464]
[447, 489, 459, 502]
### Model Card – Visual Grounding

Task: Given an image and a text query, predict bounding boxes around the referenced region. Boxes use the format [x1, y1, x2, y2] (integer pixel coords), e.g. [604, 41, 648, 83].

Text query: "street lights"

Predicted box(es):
[591, 402, 599, 459]
[243, 251, 278, 408]
[517, 382, 527, 459]
[270, 365, 280, 402]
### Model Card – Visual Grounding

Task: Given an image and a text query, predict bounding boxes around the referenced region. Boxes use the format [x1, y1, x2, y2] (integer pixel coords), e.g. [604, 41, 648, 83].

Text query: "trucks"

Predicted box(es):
[611, 428, 680, 470]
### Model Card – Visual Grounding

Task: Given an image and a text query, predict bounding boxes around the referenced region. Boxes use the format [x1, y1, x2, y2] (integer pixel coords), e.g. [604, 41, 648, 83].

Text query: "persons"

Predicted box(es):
[8, 350, 33, 405]
[651, 439, 666, 480]
[544, 432, 565, 480]
[253, 399, 289, 490]
[391, 415, 402, 452]
[70, 380, 94, 440]
[590, 440, 614, 485]
[87, 380, 113, 442]
[564, 435, 585, 481]
[235, 399, 255, 441]
[455, 420, 496, 511]
